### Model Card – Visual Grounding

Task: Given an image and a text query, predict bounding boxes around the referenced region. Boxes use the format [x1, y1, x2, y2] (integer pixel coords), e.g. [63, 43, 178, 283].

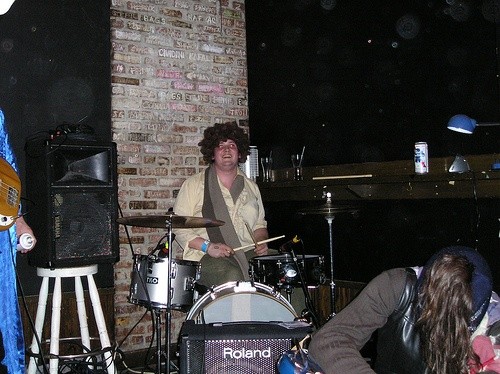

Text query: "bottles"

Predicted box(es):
[16, 232, 34, 252]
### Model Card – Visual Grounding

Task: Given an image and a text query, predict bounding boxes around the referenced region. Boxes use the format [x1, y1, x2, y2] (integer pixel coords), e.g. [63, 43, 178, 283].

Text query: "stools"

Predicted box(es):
[28, 262, 117, 374]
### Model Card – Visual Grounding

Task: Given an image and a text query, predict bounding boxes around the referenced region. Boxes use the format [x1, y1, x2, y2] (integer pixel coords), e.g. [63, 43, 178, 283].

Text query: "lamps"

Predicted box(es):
[446, 114, 500, 135]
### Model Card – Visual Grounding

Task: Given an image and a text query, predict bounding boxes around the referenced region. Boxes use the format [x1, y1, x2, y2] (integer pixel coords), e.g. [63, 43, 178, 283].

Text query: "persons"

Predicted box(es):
[171, 123, 269, 296]
[309, 246, 500, 374]
[0, 108, 36, 374]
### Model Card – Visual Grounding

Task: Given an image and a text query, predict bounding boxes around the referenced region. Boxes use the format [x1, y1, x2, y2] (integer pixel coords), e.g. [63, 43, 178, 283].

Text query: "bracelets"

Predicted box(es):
[201, 241, 210, 251]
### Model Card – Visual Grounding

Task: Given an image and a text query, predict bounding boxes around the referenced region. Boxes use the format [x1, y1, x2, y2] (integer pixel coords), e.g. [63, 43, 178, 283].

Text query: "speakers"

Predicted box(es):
[179, 318, 313, 374]
[24, 137, 120, 267]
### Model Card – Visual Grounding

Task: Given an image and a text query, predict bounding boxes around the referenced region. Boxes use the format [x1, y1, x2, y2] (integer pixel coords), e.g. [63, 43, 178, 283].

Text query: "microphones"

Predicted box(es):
[278, 234, 303, 254]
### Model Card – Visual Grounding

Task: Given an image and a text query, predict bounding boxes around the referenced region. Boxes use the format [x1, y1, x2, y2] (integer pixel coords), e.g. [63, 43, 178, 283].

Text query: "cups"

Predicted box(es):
[261, 157, 272, 182]
[291, 154, 302, 181]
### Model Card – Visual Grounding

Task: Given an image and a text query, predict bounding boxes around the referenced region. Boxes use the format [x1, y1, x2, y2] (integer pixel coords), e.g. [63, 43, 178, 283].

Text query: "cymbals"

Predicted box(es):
[117, 215, 225, 228]
[295, 203, 361, 216]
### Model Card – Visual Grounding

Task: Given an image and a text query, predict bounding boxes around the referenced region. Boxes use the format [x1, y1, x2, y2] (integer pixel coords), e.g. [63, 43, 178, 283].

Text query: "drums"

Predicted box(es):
[247, 253, 327, 288]
[186, 281, 299, 325]
[127, 253, 202, 312]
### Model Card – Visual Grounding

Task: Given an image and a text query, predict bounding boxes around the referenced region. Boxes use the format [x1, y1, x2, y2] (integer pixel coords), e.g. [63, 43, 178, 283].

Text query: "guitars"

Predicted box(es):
[0, 157, 22, 232]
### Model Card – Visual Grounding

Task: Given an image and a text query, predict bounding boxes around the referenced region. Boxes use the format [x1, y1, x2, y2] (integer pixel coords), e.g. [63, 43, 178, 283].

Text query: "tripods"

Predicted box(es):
[140, 309, 179, 374]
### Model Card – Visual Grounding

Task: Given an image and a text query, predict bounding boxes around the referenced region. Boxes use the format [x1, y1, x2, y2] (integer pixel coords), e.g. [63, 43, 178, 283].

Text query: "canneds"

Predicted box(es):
[414, 142, 428, 174]
[17, 233, 34, 252]
